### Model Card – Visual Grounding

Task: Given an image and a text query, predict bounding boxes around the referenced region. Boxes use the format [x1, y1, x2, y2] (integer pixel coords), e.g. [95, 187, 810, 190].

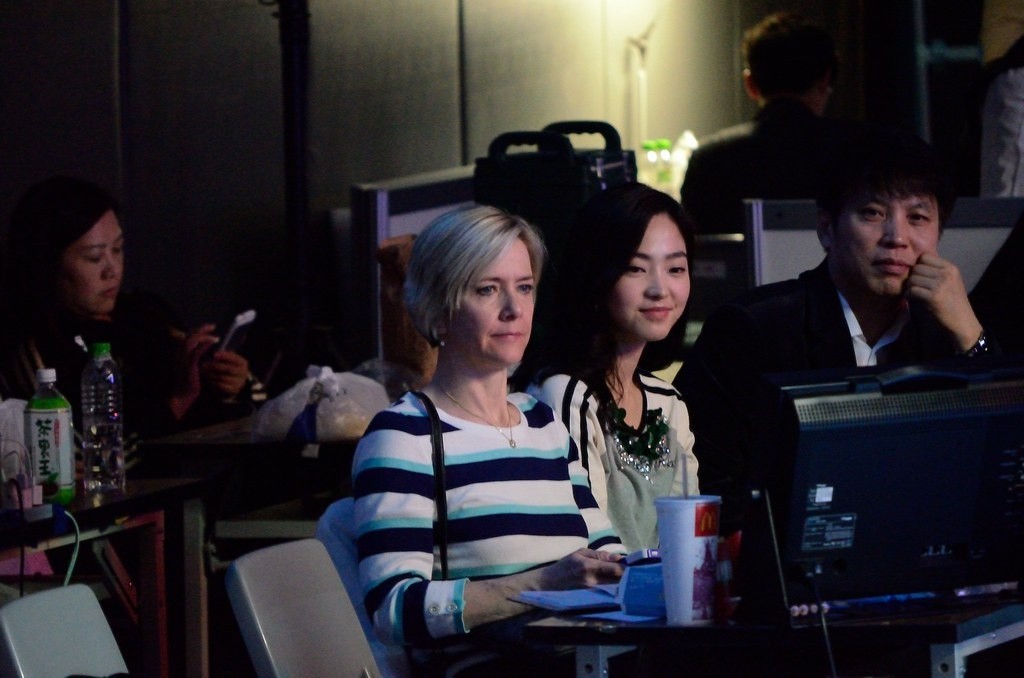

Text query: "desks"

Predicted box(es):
[0, 387, 1024, 677]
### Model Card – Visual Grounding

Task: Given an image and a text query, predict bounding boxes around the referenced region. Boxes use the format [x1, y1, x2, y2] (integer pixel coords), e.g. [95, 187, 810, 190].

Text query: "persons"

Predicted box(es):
[666, 9, 902, 230]
[975, 23, 1024, 204]
[511, 178, 732, 678]
[339, 199, 654, 678]
[0, 170, 282, 567]
[661, 131, 1024, 628]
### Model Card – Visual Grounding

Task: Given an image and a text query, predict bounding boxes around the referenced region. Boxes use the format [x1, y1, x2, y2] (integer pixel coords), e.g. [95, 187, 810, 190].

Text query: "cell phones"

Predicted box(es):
[222, 310, 257, 353]
[618, 549, 661, 567]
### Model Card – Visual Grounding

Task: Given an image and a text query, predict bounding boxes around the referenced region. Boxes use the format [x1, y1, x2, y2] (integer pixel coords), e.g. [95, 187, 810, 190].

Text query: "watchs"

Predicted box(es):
[961, 326, 989, 367]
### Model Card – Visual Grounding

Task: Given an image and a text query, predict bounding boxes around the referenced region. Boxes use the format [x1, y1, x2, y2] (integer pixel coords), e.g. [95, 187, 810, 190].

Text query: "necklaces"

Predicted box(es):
[604, 404, 675, 484]
[432, 380, 518, 448]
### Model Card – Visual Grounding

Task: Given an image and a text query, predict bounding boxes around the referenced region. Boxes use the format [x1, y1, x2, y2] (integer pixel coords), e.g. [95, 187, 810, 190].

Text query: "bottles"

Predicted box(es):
[80, 344, 128, 496]
[636, 143, 659, 188]
[655, 139, 672, 191]
[24, 368, 76, 508]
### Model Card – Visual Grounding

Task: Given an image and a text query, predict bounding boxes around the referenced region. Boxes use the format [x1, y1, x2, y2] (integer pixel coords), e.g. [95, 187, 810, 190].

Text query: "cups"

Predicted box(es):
[655, 494, 721, 626]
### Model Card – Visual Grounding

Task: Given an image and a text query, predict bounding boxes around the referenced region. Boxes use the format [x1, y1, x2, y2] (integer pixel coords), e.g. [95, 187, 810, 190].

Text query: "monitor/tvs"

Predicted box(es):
[730, 362, 1024, 619]
